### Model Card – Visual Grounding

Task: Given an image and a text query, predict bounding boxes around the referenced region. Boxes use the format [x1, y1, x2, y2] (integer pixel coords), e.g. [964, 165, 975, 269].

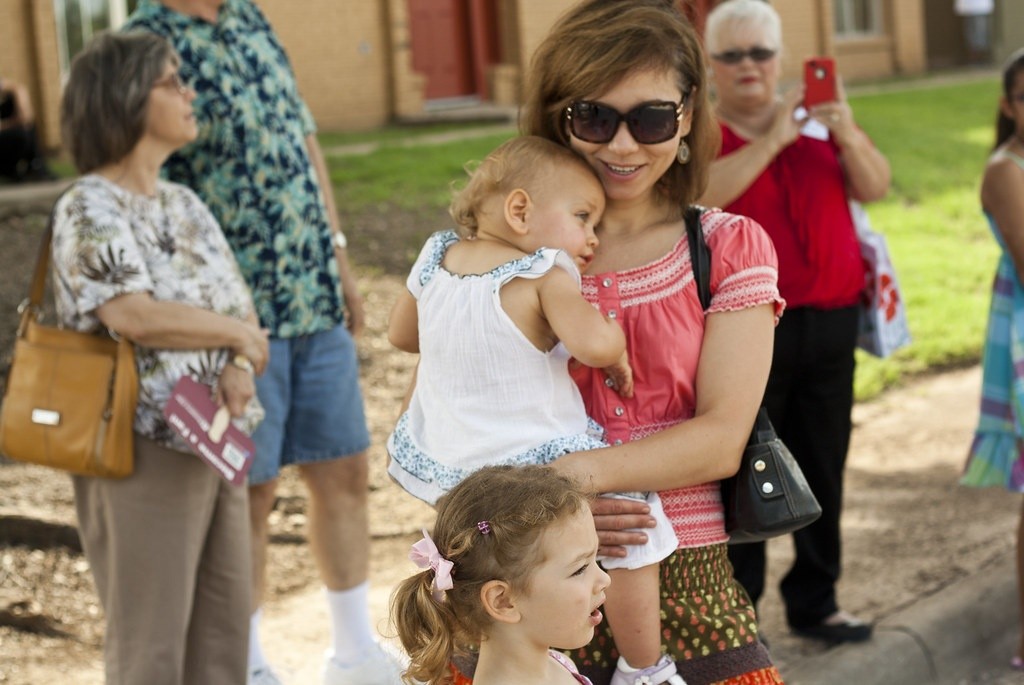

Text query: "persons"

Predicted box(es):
[49, 29, 270, 684]
[960, 48, 1024, 603]
[693, 1, 890, 650]
[387, 465, 611, 685]
[116, 0, 412, 684]
[509, 0, 786, 684]
[388, 135, 693, 685]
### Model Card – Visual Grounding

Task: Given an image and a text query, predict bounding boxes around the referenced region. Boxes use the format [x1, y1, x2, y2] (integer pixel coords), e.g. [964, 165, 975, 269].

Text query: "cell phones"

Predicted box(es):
[803, 57, 839, 107]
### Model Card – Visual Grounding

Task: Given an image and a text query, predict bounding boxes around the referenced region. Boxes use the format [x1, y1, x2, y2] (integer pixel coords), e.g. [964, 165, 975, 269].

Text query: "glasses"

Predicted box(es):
[709, 45, 775, 62]
[152, 70, 186, 96]
[564, 92, 689, 144]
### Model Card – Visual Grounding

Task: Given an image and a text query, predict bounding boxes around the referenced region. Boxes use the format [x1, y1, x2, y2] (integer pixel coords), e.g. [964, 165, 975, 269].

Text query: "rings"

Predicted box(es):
[830, 113, 839, 122]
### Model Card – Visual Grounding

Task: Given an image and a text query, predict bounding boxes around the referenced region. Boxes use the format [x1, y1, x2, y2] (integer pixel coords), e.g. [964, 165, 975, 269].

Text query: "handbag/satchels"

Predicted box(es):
[683, 205, 821, 542]
[0, 182, 138, 480]
[849, 198, 909, 357]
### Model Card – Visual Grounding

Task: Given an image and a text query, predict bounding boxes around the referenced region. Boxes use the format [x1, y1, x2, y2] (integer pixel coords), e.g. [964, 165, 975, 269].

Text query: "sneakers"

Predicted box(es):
[324, 640, 428, 685]
[246, 632, 279, 685]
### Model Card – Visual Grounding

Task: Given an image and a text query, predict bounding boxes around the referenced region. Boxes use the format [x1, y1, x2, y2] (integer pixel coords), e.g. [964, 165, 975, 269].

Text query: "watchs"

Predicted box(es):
[232, 354, 254, 376]
[331, 232, 347, 249]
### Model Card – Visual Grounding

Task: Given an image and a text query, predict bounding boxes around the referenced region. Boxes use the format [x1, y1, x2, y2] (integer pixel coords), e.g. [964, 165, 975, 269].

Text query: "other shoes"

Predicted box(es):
[610, 654, 688, 685]
[790, 611, 874, 641]
